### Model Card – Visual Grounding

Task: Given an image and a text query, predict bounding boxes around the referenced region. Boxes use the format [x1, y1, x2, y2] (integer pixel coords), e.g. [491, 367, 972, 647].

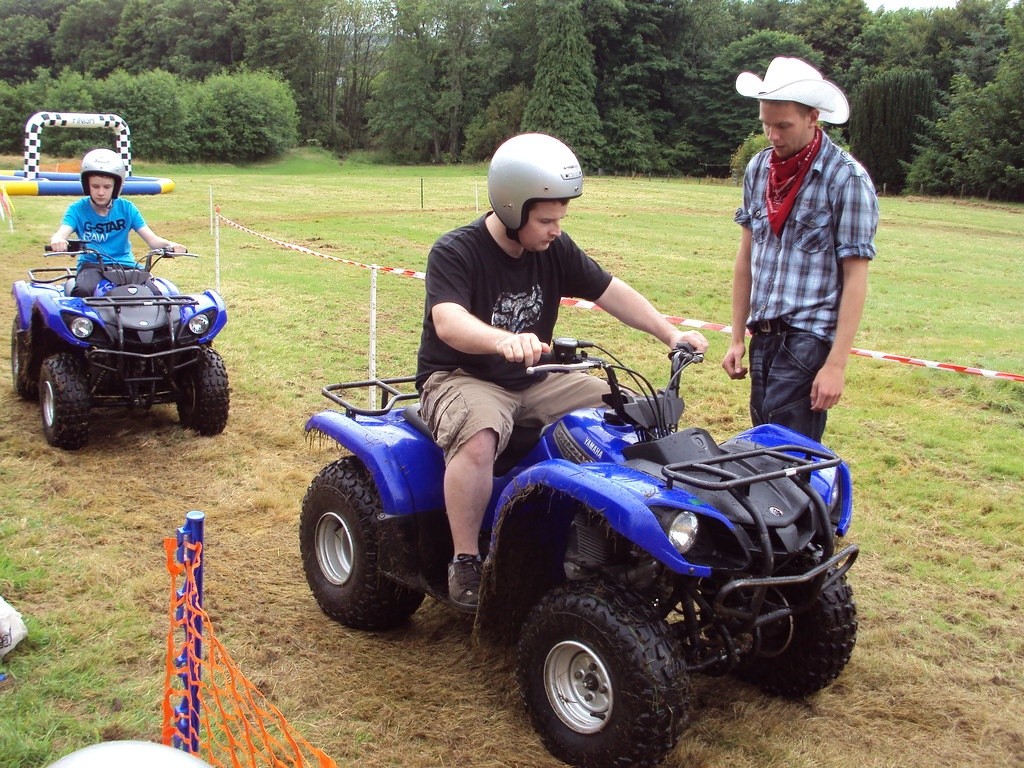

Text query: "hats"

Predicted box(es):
[736, 56, 850, 124]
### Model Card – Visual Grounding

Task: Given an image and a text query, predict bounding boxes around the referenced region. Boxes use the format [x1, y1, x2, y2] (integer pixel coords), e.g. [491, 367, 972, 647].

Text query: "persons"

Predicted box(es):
[50, 149, 187, 298]
[721, 55, 880, 443]
[414, 133, 709, 613]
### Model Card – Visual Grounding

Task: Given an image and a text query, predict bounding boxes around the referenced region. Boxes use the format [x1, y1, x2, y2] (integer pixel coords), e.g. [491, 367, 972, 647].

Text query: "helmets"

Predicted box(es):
[80, 149, 125, 200]
[488, 133, 583, 229]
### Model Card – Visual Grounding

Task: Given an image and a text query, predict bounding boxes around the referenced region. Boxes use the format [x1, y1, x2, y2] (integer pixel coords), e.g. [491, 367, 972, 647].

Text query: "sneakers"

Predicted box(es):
[447, 552, 484, 608]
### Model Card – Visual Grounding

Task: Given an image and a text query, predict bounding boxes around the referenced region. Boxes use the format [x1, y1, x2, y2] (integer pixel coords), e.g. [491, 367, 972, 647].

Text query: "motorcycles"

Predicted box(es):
[296, 336, 861, 768]
[11, 241, 231, 455]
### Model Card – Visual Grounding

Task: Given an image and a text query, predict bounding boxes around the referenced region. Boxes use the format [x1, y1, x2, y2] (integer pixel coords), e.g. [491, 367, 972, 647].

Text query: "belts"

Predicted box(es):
[753, 317, 791, 336]
